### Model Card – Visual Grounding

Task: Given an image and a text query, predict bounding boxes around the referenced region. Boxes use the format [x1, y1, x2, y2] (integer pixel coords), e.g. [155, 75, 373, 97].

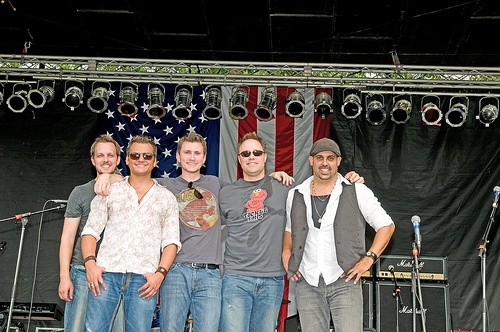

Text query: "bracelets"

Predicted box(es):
[155, 267, 168, 278]
[83, 255, 96, 267]
[365, 251, 377, 261]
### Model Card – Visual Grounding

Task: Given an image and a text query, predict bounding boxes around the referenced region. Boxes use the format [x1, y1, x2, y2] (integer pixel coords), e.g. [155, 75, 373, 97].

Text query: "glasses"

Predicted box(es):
[237, 149, 265, 157]
[188, 181, 204, 199]
[128, 153, 155, 160]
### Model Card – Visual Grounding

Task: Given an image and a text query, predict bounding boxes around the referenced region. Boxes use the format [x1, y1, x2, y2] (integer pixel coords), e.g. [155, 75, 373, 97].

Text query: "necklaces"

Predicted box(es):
[312, 181, 336, 223]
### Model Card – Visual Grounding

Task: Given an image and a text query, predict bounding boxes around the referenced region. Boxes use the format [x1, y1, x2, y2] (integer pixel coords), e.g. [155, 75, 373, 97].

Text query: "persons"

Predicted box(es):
[80, 136, 183, 332]
[218, 132, 301, 332]
[58, 135, 122, 332]
[281, 137, 395, 332]
[93, 133, 295, 332]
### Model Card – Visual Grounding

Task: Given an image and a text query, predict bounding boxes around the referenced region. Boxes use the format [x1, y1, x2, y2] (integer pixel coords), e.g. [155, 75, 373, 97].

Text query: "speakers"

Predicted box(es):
[283, 280, 451, 332]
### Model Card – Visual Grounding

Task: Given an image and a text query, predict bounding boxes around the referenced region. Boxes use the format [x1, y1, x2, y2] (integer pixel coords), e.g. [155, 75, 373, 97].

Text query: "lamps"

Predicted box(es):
[284, 86, 306, 117]
[145, 83, 166, 118]
[64, 80, 85, 108]
[87, 81, 111, 114]
[420, 94, 443, 124]
[341, 88, 362, 119]
[27, 79, 55, 108]
[445, 96, 470, 127]
[390, 93, 413, 124]
[478, 96, 499, 124]
[365, 95, 386, 126]
[172, 83, 193, 119]
[202, 85, 222, 120]
[228, 86, 250, 120]
[7, 83, 30, 112]
[117, 82, 139, 116]
[313, 87, 334, 116]
[254, 85, 278, 120]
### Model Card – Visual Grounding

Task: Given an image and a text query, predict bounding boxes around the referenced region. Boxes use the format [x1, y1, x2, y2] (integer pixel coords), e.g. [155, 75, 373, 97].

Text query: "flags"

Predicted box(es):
[99, 83, 333, 317]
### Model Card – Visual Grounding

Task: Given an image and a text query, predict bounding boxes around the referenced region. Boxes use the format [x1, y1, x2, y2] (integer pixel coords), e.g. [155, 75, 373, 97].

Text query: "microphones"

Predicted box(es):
[411, 215, 422, 248]
[387, 264, 395, 275]
[51, 199, 67, 204]
[492, 185, 500, 207]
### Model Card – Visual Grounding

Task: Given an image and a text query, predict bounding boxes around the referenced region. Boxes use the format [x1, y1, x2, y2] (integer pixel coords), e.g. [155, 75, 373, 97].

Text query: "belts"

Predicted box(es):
[190, 262, 220, 269]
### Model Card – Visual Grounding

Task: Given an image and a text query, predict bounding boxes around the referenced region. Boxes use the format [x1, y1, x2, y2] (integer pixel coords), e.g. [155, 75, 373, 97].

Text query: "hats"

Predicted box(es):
[309, 138, 341, 157]
